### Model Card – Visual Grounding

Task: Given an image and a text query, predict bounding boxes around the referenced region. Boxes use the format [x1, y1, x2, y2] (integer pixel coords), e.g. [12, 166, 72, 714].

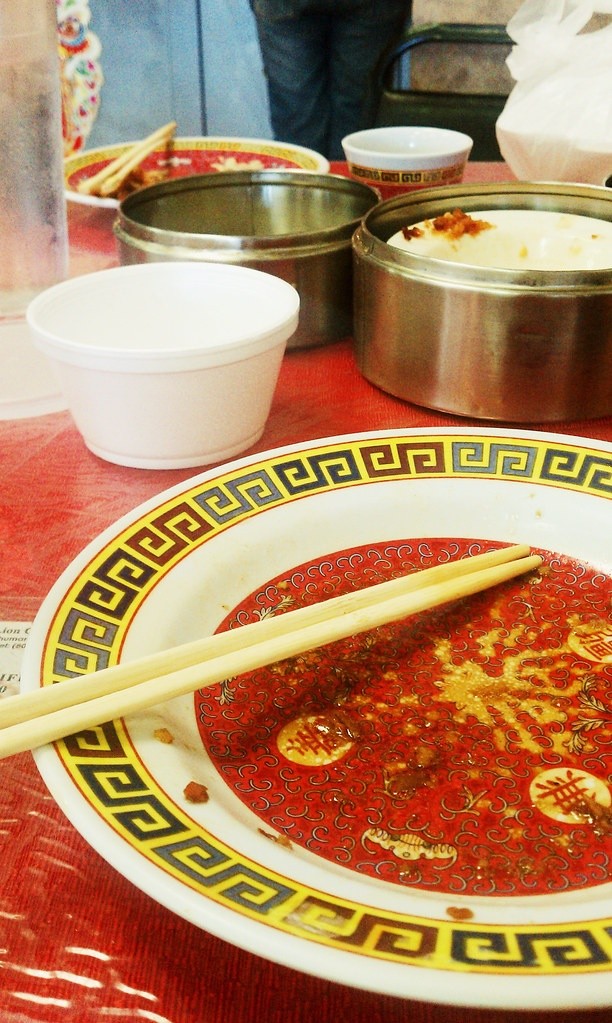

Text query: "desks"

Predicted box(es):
[0, 162, 612, 1022]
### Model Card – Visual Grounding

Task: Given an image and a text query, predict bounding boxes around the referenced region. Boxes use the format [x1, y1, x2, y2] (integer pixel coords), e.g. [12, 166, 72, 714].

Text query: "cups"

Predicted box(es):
[0, 0, 67, 322]
[341, 126, 474, 200]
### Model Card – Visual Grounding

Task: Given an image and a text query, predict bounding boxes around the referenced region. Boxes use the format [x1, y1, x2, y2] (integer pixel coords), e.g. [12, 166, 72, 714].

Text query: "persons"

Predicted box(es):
[249, 0, 415, 163]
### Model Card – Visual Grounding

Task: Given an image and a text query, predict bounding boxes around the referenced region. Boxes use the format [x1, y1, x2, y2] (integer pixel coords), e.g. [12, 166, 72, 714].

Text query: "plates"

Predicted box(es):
[20, 423, 612, 1010]
[67, 137, 329, 207]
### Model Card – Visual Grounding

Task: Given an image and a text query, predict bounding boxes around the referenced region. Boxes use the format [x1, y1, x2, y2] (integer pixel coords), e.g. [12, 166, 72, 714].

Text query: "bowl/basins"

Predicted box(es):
[111, 168, 382, 349]
[350, 180, 612, 422]
[26, 261, 301, 469]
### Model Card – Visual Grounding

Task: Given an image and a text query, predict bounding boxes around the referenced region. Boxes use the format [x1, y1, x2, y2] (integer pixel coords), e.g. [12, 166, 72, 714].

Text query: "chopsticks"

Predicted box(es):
[0, 543, 542, 758]
[77, 121, 178, 196]
